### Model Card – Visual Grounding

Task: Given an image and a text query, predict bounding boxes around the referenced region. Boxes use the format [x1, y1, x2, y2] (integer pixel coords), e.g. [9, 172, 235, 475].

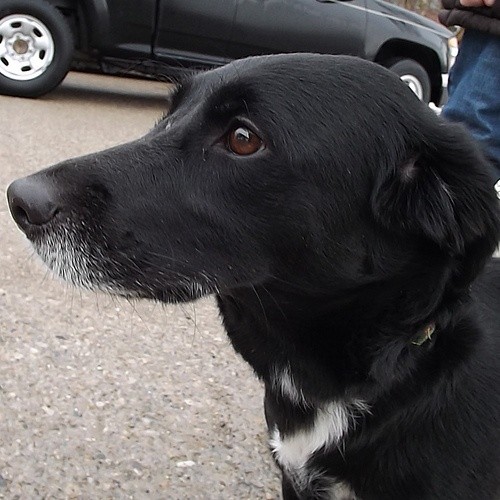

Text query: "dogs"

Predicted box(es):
[7, 53, 500, 500]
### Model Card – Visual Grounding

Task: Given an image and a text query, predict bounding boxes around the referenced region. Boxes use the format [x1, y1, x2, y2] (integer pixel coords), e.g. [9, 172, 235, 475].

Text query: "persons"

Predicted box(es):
[438, 0, 500, 186]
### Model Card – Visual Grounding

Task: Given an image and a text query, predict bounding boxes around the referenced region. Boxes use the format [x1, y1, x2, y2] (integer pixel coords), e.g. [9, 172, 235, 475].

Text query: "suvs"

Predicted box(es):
[0, 0, 462, 108]
